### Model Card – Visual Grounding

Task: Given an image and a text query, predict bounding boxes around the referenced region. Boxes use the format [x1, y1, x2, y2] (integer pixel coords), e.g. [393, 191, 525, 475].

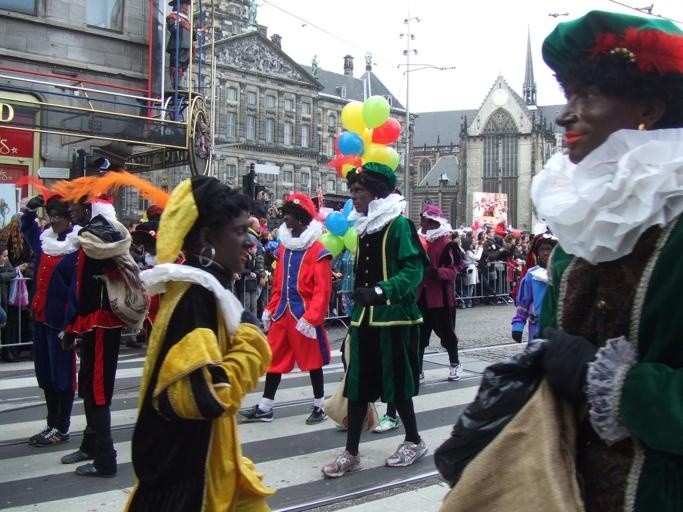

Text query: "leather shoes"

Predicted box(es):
[538, 326, 600, 404]
[510, 331, 523, 345]
[26, 192, 49, 210]
[428, 267, 437, 279]
[352, 283, 384, 307]
[239, 308, 264, 329]
[55, 330, 77, 351]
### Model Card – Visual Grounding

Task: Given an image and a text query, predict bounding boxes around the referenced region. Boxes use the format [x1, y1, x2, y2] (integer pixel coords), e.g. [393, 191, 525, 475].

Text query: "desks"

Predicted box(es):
[396, 16, 457, 220]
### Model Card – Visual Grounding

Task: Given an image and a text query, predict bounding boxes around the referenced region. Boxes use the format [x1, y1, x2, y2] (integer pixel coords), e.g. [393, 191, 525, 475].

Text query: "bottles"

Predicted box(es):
[344, 161, 396, 189]
[45, 193, 74, 218]
[512, 230, 521, 240]
[530, 232, 558, 257]
[419, 205, 446, 224]
[539, 9, 683, 80]
[493, 219, 507, 235]
[282, 191, 318, 220]
[152, 171, 233, 266]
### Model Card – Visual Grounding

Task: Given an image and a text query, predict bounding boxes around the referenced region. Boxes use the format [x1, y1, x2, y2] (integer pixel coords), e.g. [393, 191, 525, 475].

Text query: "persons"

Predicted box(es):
[127, 175, 274, 511]
[432, 12, 681, 510]
[418, 204, 463, 381]
[512, 226, 554, 307]
[321, 161, 430, 478]
[165, 1, 208, 90]
[312, 54, 321, 75]
[2, 169, 170, 478]
[241, 192, 333, 425]
[511, 234, 558, 343]
[231, 192, 535, 325]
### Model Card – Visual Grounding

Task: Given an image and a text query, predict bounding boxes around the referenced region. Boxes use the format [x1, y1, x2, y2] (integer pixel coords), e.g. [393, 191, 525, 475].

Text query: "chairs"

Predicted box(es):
[303, 405, 328, 425]
[120, 336, 146, 351]
[27, 428, 49, 445]
[37, 427, 69, 446]
[384, 437, 429, 468]
[236, 403, 274, 422]
[0, 347, 34, 363]
[73, 462, 116, 478]
[372, 414, 399, 432]
[455, 295, 515, 309]
[59, 449, 93, 464]
[320, 449, 361, 477]
[447, 363, 462, 381]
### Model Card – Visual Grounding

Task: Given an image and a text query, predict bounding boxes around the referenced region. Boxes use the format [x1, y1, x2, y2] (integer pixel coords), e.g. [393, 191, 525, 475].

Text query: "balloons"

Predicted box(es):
[318, 96, 401, 259]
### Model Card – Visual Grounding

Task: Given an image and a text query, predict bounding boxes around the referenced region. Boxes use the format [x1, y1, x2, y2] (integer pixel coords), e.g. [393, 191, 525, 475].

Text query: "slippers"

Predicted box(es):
[75, 217, 152, 334]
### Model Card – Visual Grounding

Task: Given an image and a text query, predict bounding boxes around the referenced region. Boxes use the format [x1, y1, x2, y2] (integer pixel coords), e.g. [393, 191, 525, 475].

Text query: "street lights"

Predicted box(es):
[320, 332, 382, 434]
[435, 376, 590, 512]
